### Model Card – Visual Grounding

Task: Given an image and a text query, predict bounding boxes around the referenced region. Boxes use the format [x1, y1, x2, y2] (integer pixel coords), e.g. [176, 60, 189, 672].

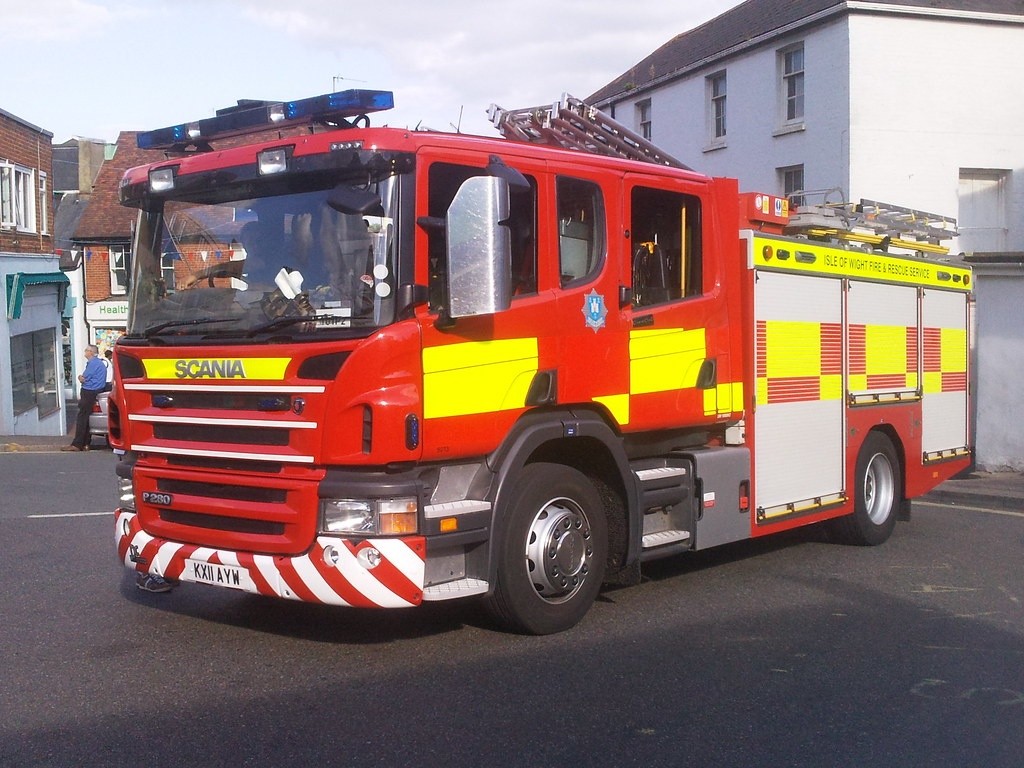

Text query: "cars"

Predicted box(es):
[90, 387, 111, 437]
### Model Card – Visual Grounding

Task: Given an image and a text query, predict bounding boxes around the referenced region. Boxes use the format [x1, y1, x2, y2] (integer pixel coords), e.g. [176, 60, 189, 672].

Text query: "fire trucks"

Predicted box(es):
[109, 88, 972, 636]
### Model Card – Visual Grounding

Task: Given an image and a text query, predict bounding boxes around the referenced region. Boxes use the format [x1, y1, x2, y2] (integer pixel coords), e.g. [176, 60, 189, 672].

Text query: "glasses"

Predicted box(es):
[85, 349, 93, 353]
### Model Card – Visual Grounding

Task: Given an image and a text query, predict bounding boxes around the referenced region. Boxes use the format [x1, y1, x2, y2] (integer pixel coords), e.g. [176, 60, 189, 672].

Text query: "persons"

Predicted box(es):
[171, 220, 292, 291]
[101, 350, 113, 392]
[61, 345, 107, 451]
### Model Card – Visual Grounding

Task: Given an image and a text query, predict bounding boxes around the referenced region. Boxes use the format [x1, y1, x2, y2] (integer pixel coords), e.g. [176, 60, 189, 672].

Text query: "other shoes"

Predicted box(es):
[61, 444, 82, 452]
[81, 445, 90, 452]
